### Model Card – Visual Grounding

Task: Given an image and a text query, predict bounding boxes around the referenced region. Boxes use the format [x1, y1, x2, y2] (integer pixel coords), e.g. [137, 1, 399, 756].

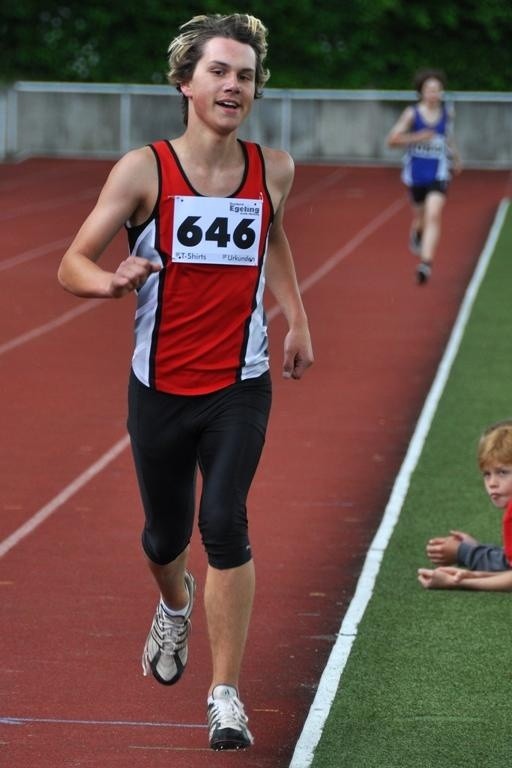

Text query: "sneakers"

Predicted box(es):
[408, 228, 434, 285]
[141, 568, 196, 687]
[205, 684, 250, 751]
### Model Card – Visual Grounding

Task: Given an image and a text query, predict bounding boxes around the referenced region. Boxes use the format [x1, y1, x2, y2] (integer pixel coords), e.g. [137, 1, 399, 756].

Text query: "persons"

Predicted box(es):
[417, 422, 512, 592]
[387, 72, 459, 284]
[57, 14, 315, 750]
